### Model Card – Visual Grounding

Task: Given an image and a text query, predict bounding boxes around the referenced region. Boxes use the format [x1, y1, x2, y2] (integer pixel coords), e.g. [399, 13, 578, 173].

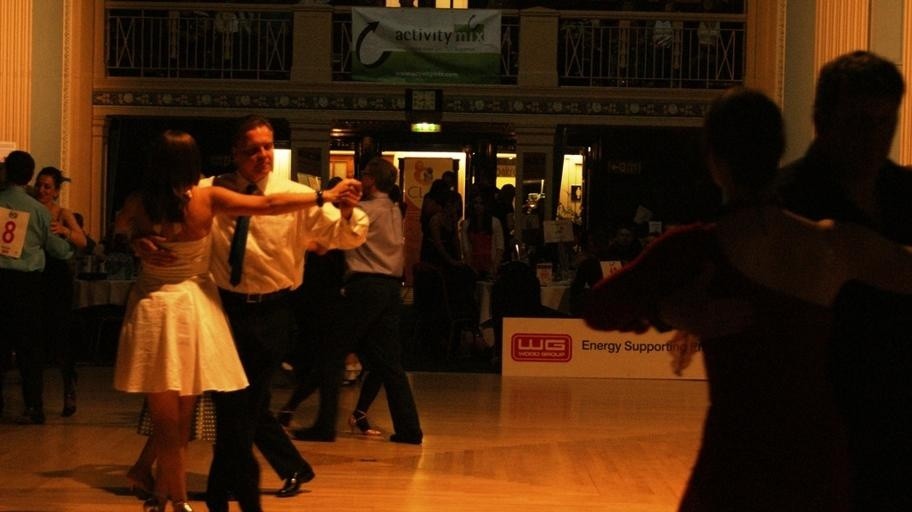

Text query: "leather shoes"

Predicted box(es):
[30, 407, 46, 423]
[391, 434, 422, 444]
[278, 461, 315, 495]
[288, 426, 336, 441]
[63, 390, 77, 417]
[127, 462, 192, 512]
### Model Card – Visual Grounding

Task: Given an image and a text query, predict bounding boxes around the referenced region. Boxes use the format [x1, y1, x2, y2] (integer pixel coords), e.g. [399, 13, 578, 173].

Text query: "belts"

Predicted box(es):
[218, 287, 292, 303]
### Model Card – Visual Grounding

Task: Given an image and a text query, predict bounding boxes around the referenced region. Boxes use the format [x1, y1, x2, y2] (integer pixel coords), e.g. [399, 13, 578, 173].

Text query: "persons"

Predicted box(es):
[24, 166, 90, 417]
[125, 114, 371, 511]
[124, 387, 316, 501]
[648, 48, 912, 510]
[401, 166, 615, 372]
[584, 74, 910, 511]
[271, 174, 385, 441]
[110, 128, 364, 511]
[1, 150, 73, 425]
[74, 208, 141, 282]
[292, 157, 424, 444]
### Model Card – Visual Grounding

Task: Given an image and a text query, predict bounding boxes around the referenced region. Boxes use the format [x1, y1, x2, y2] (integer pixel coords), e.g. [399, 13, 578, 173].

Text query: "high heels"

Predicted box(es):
[349, 415, 381, 435]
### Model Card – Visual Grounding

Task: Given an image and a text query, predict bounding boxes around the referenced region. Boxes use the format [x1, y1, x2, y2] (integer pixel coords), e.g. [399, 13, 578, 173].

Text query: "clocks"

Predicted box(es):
[405, 88, 443, 118]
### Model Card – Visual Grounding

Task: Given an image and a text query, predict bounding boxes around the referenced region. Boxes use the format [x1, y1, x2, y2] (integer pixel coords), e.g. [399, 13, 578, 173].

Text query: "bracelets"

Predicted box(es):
[65, 229, 73, 241]
[316, 191, 324, 208]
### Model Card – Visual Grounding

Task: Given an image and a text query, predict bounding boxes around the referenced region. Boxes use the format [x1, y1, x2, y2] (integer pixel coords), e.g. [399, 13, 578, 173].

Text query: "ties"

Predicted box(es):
[230, 182, 257, 287]
